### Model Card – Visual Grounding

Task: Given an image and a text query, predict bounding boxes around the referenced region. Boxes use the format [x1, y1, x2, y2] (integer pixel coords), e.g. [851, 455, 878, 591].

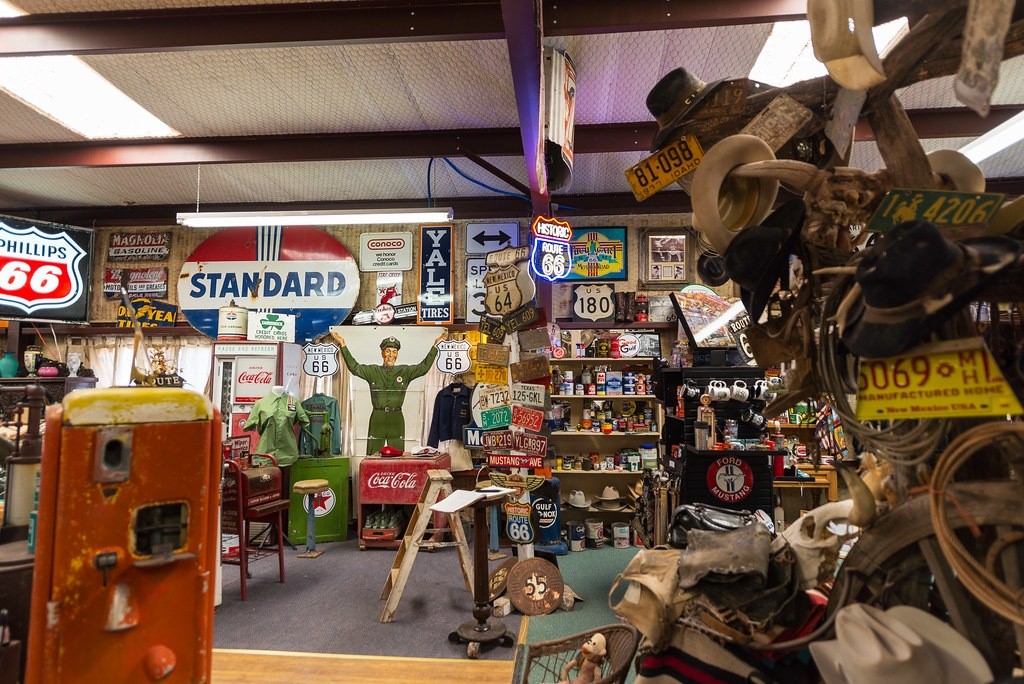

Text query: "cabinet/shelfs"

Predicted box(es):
[765, 421, 836, 511]
[541, 355, 662, 532]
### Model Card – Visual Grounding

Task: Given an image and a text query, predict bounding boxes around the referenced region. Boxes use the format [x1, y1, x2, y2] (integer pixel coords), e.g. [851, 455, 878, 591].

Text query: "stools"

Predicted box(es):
[291, 479, 329, 560]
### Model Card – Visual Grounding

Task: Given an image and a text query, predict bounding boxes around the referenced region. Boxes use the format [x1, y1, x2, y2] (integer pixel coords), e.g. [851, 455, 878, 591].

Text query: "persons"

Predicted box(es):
[243, 385, 310, 467]
[332, 330, 445, 455]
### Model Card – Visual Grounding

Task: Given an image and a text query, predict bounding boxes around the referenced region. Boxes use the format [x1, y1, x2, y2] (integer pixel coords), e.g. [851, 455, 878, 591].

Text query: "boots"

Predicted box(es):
[615, 292, 625, 322]
[626, 292, 635, 322]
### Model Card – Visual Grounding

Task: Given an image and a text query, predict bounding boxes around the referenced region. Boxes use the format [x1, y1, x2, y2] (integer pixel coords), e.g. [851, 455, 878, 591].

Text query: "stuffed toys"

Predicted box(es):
[557, 633, 607, 684]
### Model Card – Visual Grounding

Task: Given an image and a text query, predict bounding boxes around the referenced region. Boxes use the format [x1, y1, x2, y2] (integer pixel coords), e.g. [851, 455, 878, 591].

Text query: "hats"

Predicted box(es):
[595, 485, 627, 500]
[837, 221, 1023, 358]
[593, 501, 627, 511]
[808, 602, 994, 684]
[697, 250, 730, 287]
[692, 135, 780, 255]
[646, 67, 731, 153]
[625, 478, 643, 510]
[724, 200, 805, 328]
[927, 150, 986, 193]
[561, 490, 591, 512]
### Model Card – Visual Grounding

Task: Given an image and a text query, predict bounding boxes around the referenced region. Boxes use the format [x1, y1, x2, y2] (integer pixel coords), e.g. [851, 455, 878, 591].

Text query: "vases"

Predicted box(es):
[0, 350, 20, 379]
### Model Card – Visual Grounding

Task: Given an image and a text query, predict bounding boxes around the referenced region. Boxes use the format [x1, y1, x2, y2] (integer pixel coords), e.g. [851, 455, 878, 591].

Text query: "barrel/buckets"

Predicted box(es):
[217, 308, 248, 340]
[584, 518, 603, 549]
[566, 521, 585, 551]
[611, 522, 630, 548]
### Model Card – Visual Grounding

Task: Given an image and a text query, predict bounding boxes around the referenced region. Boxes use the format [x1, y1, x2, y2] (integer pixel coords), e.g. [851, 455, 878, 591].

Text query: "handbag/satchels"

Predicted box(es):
[666, 502, 776, 548]
[609, 548, 851, 684]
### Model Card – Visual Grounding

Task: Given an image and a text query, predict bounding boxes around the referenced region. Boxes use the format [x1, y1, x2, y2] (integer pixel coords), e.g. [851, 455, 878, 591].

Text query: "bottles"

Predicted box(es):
[0, 352, 19, 378]
[724, 418, 738, 440]
[365, 510, 402, 529]
[778, 359, 803, 392]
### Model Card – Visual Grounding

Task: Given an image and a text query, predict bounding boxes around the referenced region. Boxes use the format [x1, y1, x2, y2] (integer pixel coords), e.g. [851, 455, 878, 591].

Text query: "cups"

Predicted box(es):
[67, 353, 82, 377]
[693, 421, 712, 451]
[793, 444, 807, 456]
[25, 351, 43, 378]
[679, 377, 783, 402]
[740, 405, 766, 427]
[790, 414, 802, 424]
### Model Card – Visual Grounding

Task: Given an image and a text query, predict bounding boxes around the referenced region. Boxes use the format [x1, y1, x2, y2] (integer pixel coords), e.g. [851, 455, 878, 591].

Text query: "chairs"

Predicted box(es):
[522, 624, 638, 684]
[224, 450, 285, 601]
